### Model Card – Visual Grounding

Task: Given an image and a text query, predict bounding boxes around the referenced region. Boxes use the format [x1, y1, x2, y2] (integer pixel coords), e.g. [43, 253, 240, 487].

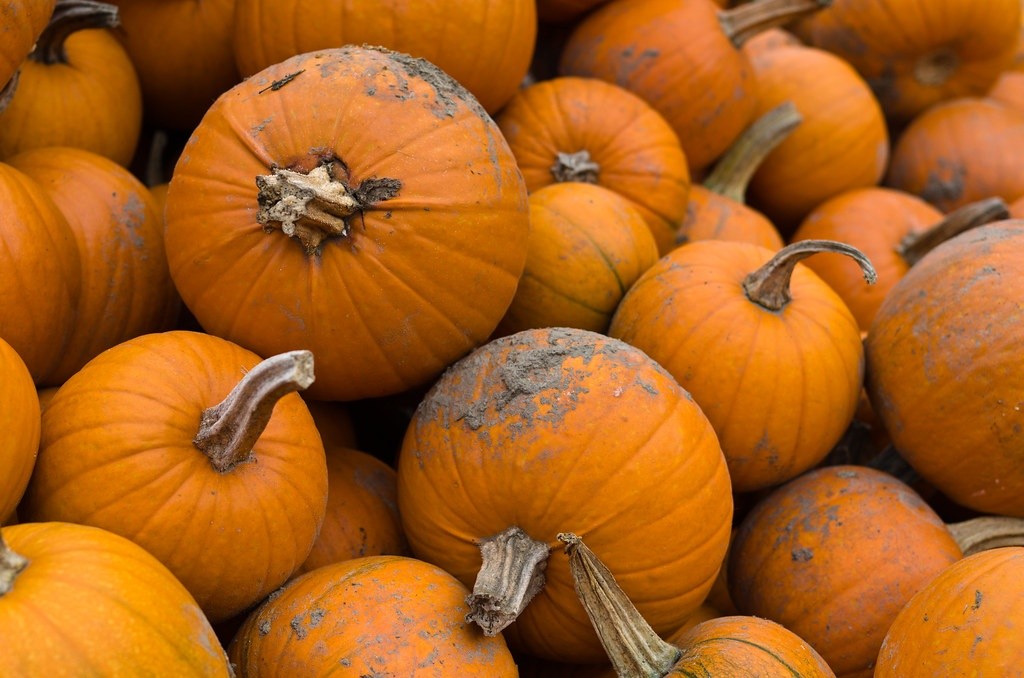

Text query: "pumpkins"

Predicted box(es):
[0, 0, 1024, 678]
